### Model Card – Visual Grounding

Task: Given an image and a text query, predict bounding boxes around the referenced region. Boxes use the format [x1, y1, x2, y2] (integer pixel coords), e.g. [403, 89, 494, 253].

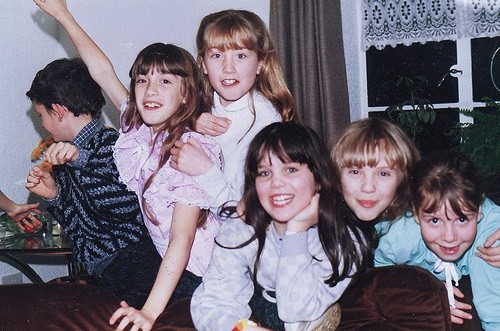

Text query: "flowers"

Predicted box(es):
[31, 137, 54, 172]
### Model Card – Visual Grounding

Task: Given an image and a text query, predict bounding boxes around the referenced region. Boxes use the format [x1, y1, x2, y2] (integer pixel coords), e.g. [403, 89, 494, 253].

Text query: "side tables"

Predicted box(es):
[0, 233, 77, 283]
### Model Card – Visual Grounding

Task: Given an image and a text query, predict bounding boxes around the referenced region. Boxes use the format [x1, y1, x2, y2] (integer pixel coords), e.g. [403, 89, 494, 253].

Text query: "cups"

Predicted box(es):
[0, 220, 16, 249]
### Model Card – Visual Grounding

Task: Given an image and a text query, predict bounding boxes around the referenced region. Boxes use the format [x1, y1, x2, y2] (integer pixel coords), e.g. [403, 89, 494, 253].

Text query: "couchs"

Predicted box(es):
[0, 265, 483, 331]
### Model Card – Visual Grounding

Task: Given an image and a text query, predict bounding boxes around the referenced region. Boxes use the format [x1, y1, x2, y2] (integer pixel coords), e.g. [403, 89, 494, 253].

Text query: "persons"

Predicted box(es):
[0, 56, 500, 331]
[34, 1, 226, 331]
[170, 9, 284, 222]
